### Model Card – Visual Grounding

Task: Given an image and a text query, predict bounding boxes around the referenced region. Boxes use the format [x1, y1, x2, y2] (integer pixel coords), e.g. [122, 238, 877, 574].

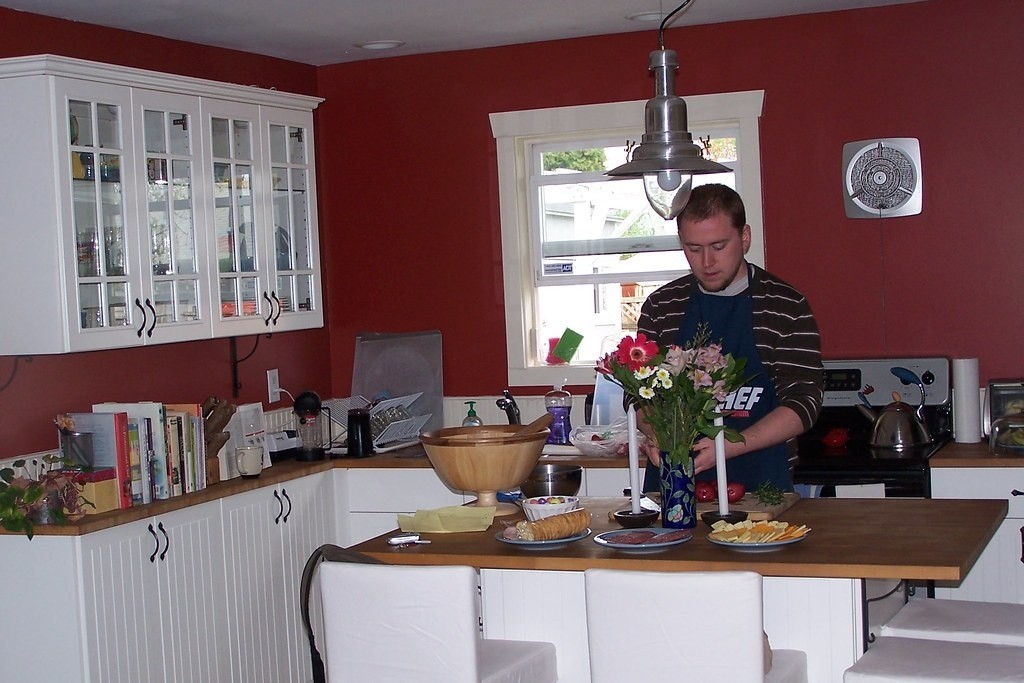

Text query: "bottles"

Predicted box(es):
[346, 408, 374, 459]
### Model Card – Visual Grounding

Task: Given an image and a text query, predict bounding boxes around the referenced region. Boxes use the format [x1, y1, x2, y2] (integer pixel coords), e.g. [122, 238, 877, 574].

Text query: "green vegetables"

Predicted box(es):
[752, 479, 784, 507]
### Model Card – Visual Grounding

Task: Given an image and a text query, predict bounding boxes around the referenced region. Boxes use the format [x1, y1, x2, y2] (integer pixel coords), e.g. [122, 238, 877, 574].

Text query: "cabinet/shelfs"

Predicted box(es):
[0, 470, 337, 683]
[338, 468, 1024, 604]
[0, 54, 325, 356]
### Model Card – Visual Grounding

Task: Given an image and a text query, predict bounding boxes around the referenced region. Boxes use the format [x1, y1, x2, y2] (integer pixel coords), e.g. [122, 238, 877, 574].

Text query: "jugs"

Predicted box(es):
[291, 390, 332, 461]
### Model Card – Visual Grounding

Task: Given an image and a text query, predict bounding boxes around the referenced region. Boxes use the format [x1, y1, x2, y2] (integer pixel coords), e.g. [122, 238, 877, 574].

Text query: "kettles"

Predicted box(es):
[855, 367, 930, 448]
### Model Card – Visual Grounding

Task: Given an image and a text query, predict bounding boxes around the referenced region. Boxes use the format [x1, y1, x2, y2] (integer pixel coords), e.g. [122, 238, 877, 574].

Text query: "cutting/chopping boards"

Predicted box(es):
[608, 491, 800, 521]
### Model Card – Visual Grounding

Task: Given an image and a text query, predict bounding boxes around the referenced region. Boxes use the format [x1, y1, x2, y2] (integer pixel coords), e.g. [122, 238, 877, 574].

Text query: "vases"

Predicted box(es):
[658, 450, 697, 528]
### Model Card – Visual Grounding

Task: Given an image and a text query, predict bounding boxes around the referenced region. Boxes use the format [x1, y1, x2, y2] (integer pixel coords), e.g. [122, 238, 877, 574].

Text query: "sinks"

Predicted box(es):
[542, 444, 578, 454]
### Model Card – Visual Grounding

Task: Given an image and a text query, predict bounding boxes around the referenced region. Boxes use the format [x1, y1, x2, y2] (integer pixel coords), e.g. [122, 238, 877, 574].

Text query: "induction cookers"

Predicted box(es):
[793, 357, 950, 474]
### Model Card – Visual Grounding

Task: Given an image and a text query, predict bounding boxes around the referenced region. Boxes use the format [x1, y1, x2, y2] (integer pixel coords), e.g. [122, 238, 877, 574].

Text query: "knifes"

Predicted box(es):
[623, 487, 662, 513]
[202, 396, 237, 458]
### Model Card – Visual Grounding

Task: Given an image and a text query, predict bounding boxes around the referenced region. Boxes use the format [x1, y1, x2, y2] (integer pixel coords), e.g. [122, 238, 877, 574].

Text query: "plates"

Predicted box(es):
[221, 297, 292, 317]
[494, 527, 592, 551]
[594, 527, 693, 555]
[706, 530, 809, 552]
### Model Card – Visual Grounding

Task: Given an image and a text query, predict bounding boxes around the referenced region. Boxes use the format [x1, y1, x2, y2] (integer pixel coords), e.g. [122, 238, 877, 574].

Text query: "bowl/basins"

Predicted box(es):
[520, 462, 583, 497]
[522, 496, 580, 521]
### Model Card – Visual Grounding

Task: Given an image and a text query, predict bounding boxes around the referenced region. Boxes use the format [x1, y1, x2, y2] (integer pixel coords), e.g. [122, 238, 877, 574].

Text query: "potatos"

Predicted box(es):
[693, 479, 745, 502]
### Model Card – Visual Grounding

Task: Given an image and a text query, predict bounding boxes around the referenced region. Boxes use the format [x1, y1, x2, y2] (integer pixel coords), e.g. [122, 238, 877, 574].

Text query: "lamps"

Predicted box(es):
[603, 0, 735, 221]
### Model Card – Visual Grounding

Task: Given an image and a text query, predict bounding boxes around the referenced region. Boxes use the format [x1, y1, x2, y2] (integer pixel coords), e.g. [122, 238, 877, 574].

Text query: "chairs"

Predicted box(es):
[842, 636, 1024, 683]
[318, 560, 559, 683]
[880, 597, 1024, 647]
[584, 567, 808, 683]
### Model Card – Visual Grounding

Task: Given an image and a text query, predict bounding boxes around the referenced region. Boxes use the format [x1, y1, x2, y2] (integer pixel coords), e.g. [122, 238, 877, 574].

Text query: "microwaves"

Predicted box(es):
[987, 381, 1024, 458]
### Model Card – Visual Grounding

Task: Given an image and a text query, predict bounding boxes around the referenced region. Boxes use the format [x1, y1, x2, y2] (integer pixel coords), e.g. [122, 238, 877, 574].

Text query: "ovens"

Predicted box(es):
[791, 473, 931, 499]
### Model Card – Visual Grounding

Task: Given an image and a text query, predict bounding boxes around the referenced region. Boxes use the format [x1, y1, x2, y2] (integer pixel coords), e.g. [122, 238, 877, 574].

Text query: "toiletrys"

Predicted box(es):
[461, 401, 483, 426]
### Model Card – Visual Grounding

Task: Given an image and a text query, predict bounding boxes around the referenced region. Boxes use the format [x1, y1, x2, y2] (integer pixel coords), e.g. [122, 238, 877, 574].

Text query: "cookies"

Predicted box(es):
[709, 519, 812, 542]
[515, 508, 591, 541]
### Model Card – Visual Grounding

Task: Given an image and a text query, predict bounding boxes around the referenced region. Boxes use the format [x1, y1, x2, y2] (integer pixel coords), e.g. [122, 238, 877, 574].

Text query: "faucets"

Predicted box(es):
[496, 390, 522, 425]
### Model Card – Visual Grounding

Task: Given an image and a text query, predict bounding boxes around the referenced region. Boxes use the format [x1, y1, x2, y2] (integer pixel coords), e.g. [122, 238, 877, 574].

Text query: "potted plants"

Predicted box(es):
[0, 453, 97, 542]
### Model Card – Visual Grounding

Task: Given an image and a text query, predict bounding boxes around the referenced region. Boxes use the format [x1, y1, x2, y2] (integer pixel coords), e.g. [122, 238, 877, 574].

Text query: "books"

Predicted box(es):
[57, 401, 206, 508]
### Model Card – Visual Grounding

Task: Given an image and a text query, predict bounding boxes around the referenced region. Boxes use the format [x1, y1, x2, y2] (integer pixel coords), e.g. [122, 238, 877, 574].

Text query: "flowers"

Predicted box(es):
[593, 321, 758, 469]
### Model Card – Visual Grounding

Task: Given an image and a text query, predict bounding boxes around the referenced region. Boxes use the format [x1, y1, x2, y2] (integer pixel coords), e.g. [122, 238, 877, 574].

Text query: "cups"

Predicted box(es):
[235, 447, 263, 479]
[62, 432, 94, 473]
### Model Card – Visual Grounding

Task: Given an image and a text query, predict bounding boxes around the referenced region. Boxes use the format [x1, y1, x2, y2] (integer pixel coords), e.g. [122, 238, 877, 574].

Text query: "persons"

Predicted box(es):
[623, 183, 826, 496]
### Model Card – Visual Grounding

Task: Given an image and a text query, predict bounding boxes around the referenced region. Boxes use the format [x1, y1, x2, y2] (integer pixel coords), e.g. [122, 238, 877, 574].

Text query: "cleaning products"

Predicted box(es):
[543, 377, 574, 444]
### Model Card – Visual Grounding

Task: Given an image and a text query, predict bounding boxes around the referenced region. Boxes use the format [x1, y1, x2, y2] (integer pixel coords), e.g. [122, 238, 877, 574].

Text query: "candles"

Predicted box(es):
[713, 404, 732, 514]
[627, 404, 642, 514]
